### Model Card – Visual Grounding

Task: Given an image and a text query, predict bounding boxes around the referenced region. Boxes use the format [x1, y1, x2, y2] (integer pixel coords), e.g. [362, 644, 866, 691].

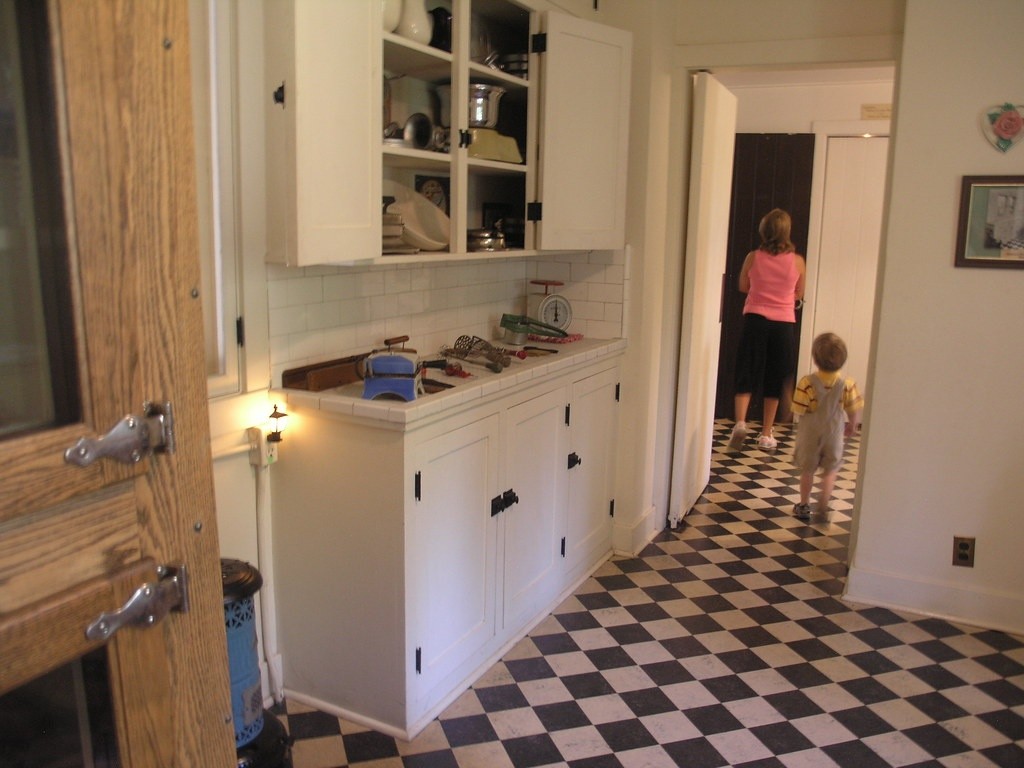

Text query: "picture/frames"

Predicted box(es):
[952, 175, 1024, 270]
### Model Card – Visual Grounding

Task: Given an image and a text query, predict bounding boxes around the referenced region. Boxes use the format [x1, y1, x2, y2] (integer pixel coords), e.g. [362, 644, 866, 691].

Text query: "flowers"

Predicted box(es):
[982, 103, 1024, 151]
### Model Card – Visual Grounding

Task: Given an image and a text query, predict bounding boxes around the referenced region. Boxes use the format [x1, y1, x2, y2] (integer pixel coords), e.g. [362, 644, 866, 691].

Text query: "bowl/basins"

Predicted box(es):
[436, 83, 506, 128]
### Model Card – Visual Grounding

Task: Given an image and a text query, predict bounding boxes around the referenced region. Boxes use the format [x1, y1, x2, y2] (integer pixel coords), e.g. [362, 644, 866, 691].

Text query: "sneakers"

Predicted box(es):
[756, 427, 777, 450]
[727, 421, 746, 451]
[816, 509, 828, 522]
[793, 503, 810, 519]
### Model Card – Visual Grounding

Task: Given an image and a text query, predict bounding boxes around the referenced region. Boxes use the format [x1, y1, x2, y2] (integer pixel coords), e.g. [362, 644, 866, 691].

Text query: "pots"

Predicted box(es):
[467, 226, 506, 251]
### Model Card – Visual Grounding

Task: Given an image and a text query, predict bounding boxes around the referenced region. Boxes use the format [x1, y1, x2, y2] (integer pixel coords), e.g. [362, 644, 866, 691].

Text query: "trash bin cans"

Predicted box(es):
[219, 557, 265, 750]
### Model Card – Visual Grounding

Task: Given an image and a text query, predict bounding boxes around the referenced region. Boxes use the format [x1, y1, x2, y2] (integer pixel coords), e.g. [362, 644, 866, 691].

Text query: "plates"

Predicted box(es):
[382, 180, 450, 251]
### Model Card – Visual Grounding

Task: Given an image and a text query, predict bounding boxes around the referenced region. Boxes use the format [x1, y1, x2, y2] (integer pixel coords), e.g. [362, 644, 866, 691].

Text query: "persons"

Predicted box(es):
[728, 209, 806, 449]
[790, 333, 864, 518]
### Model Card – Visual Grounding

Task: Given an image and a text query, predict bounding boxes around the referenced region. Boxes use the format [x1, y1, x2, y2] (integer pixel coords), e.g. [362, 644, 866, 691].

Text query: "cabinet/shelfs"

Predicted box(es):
[262, 1, 634, 269]
[269, 340, 629, 741]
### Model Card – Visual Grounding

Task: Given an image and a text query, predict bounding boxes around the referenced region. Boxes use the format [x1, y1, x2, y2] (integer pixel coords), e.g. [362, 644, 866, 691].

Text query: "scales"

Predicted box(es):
[526, 279, 573, 336]
